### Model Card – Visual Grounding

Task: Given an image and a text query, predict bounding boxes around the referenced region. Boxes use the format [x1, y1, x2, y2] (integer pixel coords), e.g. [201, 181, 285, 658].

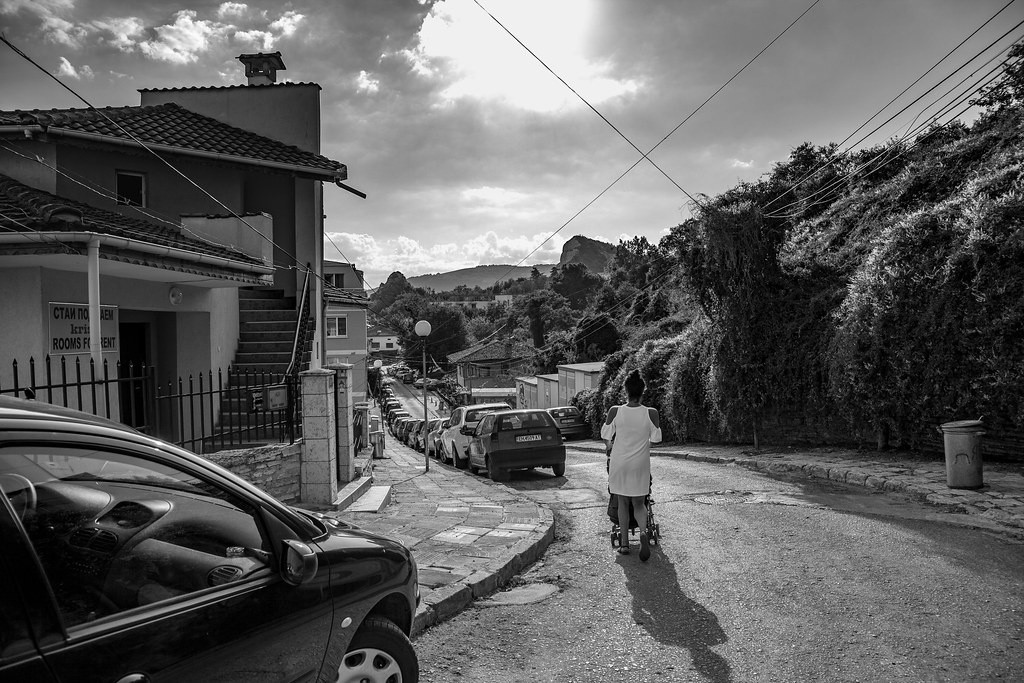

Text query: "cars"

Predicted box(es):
[387, 365, 437, 390]
[549, 406, 586, 440]
[380, 384, 451, 459]
[0, 393, 420, 683]
[464, 409, 566, 480]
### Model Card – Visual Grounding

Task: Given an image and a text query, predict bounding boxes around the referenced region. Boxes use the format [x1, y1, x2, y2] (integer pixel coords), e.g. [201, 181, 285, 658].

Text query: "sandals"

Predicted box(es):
[617, 546, 630, 555]
[639, 533, 650, 562]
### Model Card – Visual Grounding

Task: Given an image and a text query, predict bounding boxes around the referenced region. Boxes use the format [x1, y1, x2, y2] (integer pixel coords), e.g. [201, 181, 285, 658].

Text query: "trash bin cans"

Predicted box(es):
[935, 420, 987, 489]
[370, 415, 378, 431]
[439, 402, 444, 410]
[369, 431, 385, 458]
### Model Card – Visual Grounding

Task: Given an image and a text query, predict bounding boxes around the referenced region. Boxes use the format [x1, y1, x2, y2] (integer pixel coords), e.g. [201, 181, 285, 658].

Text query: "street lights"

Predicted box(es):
[374, 359, 386, 449]
[415, 320, 432, 471]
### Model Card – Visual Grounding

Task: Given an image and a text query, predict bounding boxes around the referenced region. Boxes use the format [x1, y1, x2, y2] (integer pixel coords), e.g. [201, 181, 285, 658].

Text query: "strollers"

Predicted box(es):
[607, 445, 659, 546]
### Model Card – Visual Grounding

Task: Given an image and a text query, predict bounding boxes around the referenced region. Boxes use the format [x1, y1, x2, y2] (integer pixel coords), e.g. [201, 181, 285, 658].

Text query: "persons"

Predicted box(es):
[600, 370, 662, 561]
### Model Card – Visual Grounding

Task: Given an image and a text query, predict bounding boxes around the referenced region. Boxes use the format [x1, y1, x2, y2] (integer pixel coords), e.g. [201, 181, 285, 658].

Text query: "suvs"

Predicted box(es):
[440, 402, 523, 470]
